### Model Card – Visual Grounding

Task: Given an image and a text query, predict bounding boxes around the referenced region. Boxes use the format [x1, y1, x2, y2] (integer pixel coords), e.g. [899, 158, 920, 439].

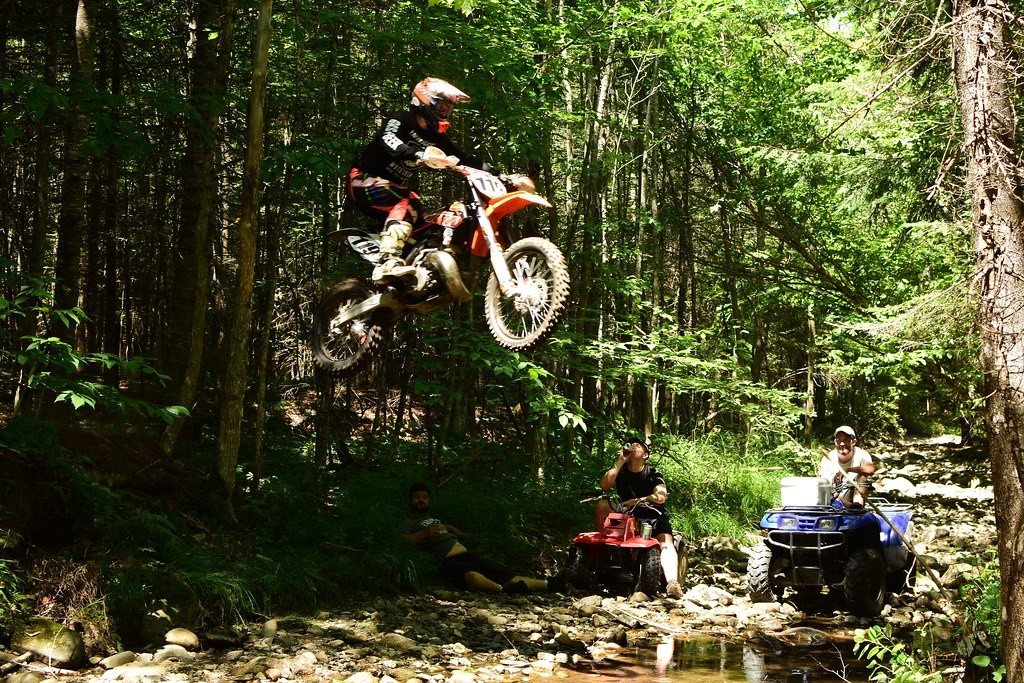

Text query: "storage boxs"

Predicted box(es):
[781, 477, 820, 507]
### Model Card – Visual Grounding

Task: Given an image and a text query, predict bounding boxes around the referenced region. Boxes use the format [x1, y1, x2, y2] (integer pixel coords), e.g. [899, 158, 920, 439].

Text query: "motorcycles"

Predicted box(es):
[567, 490, 688, 598]
[310, 148, 572, 376]
[745, 482, 917, 619]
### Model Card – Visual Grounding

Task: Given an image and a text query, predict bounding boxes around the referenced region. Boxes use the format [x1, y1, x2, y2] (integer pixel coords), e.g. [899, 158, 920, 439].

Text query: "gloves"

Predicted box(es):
[424, 146, 447, 170]
[506, 173, 535, 194]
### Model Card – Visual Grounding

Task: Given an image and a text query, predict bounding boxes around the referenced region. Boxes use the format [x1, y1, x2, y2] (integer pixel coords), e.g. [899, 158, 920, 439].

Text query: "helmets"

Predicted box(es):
[410, 77, 471, 134]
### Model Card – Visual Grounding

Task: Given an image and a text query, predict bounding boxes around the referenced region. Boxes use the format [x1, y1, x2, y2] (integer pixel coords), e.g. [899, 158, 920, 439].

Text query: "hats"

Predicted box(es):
[834, 426, 855, 438]
[628, 437, 649, 460]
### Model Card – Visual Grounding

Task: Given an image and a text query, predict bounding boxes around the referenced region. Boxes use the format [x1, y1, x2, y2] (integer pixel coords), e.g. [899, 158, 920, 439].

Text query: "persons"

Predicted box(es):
[822, 426, 875, 507]
[393, 481, 565, 593]
[350, 77, 508, 281]
[600, 438, 683, 598]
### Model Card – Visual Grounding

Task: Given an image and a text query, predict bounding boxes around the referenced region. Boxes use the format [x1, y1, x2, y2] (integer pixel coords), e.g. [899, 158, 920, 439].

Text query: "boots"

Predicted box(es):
[372, 218, 416, 284]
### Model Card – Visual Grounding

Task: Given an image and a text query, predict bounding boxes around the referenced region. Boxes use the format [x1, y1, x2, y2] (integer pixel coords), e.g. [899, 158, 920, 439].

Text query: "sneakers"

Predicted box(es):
[666, 580, 683, 598]
[501, 580, 529, 594]
[547, 569, 568, 592]
